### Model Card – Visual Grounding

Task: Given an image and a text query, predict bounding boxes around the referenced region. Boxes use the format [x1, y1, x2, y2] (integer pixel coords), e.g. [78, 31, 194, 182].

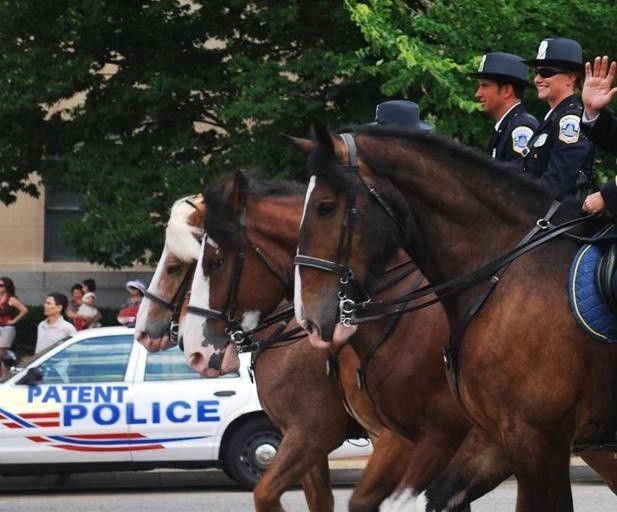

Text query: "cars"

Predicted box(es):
[0, 326, 374, 492]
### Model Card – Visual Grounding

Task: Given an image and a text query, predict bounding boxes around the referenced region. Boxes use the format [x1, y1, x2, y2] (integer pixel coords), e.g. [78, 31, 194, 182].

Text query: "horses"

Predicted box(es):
[280, 112, 617, 512]
[134, 193, 370, 512]
[178, 168, 471, 512]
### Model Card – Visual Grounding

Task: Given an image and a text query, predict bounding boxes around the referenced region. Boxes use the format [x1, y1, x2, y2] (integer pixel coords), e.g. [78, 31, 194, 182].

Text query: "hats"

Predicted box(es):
[465, 51, 533, 89]
[520, 38, 583, 68]
[126, 279, 146, 295]
[365, 99, 435, 132]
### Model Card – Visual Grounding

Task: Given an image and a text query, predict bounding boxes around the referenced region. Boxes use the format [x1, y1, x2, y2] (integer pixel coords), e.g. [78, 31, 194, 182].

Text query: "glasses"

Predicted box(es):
[534, 68, 563, 78]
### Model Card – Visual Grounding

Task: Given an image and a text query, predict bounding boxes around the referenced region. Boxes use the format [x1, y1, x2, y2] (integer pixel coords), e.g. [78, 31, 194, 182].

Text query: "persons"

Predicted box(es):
[120, 280, 145, 308]
[34, 293, 77, 356]
[465, 52, 539, 163]
[74, 279, 99, 330]
[64, 284, 102, 331]
[0, 275, 28, 378]
[579, 54, 617, 217]
[512, 36, 593, 203]
